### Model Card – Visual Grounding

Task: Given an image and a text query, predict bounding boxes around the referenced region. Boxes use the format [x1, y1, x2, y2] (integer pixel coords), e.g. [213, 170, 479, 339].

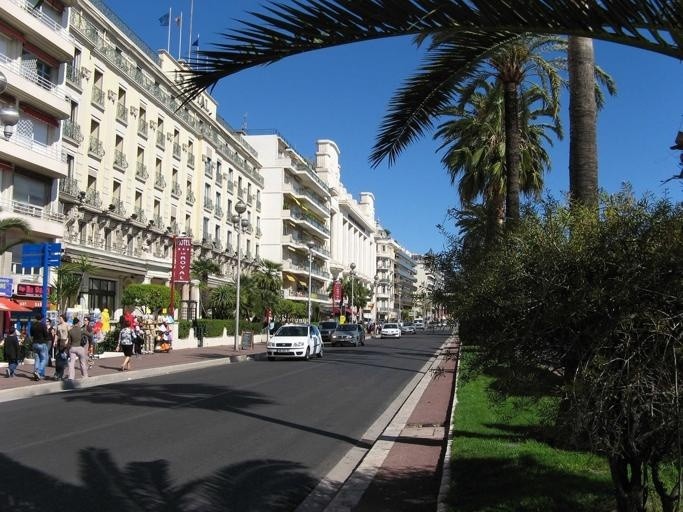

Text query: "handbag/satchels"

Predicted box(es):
[132, 335, 144, 345]
[80, 336, 86, 347]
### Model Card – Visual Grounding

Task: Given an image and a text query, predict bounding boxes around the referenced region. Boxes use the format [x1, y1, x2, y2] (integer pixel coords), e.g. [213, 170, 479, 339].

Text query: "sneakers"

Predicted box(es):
[34, 372, 40, 380]
[6, 369, 10, 378]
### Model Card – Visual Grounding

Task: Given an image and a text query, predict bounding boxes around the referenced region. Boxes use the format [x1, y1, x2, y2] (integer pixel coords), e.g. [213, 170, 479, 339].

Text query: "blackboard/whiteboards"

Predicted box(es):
[240, 330, 253, 345]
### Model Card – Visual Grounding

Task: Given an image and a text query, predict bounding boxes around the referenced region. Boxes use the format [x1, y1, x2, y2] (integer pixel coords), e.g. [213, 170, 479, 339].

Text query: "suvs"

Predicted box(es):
[267, 321, 324, 361]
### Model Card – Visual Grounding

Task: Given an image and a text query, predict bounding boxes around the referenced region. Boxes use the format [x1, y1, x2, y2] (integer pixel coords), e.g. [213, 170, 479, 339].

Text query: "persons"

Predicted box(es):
[0, 305, 175, 386]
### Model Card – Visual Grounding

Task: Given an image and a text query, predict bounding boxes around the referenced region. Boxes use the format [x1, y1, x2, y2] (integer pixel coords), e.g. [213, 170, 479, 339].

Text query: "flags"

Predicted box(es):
[158, 14, 169, 27]
[173, 16, 180, 26]
[191, 39, 198, 46]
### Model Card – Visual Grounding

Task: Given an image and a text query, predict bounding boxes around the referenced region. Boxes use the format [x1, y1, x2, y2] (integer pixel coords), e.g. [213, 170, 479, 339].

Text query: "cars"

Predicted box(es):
[401, 317, 455, 335]
[317, 321, 339, 342]
[330, 322, 365, 347]
[380, 323, 401, 338]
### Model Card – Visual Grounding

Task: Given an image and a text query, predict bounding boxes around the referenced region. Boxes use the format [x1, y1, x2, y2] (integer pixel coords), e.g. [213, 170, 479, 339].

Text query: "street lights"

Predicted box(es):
[398, 288, 402, 322]
[306, 240, 316, 323]
[374, 275, 381, 335]
[350, 261, 357, 322]
[231, 199, 249, 353]
